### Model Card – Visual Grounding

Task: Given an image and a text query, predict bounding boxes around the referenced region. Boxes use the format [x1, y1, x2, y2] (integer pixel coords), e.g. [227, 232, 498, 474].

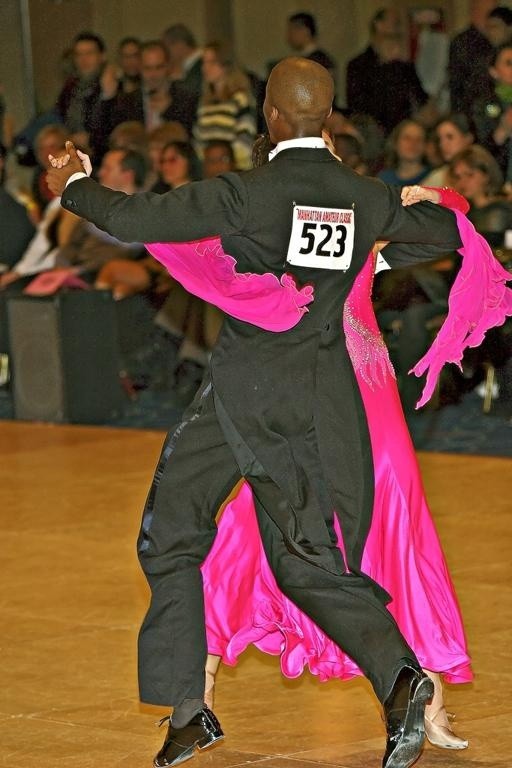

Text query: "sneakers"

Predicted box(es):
[421, 705, 469, 750]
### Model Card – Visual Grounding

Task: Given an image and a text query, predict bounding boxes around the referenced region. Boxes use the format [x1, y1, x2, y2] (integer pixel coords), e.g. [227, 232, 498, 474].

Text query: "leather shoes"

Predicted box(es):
[380, 665, 434, 768]
[153, 708, 224, 768]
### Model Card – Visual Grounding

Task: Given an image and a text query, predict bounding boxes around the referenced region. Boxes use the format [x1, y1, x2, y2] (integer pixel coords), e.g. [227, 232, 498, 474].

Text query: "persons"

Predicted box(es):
[0, 1, 335, 299]
[337, 4, 511, 421]
[45, 56, 466, 768]
[48, 126, 512, 752]
